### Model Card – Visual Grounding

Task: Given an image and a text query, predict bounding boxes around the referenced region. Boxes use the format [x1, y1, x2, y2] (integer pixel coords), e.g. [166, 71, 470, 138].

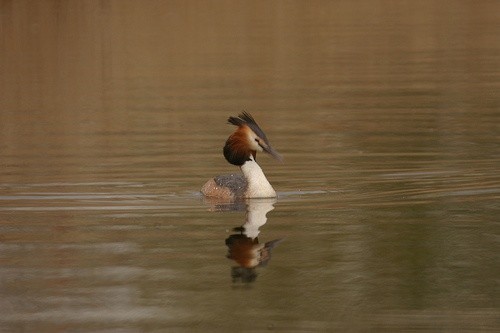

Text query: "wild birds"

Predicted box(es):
[197, 199, 284, 287]
[199, 112, 282, 200]
